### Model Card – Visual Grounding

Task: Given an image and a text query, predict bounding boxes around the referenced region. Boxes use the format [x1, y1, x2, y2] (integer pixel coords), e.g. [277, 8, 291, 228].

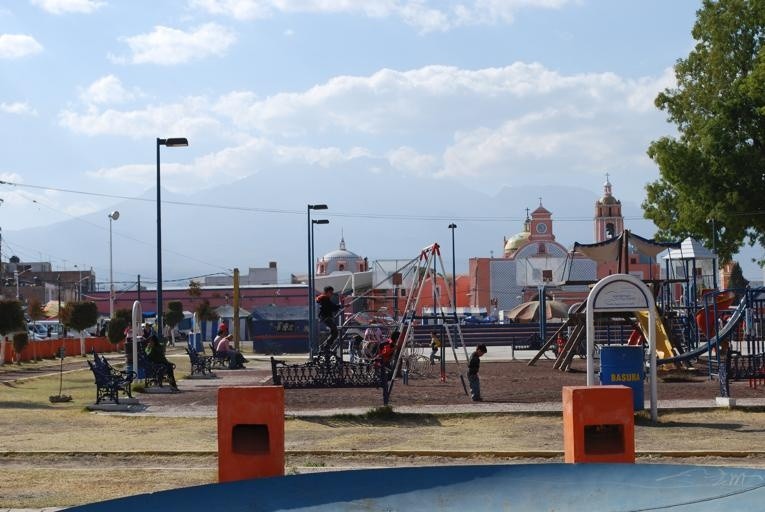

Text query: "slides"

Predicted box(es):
[694, 288, 737, 355]
[628, 306, 685, 371]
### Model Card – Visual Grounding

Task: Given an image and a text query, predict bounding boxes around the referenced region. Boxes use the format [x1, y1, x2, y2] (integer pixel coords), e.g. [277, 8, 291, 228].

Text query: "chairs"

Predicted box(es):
[86, 340, 176, 406]
[184, 340, 231, 378]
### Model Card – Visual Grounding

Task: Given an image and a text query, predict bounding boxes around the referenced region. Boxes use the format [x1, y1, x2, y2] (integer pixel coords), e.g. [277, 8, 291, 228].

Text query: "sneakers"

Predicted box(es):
[318, 344, 329, 350]
[228, 357, 249, 370]
[430, 362, 435, 365]
[438, 356, 441, 361]
[171, 387, 180, 393]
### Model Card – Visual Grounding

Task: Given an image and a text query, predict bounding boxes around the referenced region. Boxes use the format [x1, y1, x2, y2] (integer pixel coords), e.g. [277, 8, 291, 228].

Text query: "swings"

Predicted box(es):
[317, 251, 429, 326]
[371, 251, 430, 374]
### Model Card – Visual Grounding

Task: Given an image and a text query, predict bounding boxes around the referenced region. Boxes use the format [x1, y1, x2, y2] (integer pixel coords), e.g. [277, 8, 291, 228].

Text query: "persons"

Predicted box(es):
[124, 319, 174, 346]
[373, 329, 401, 389]
[213, 328, 249, 363]
[466, 343, 487, 402]
[219, 320, 227, 336]
[48, 326, 54, 338]
[315, 286, 351, 349]
[100, 324, 107, 336]
[557, 333, 567, 358]
[364, 319, 384, 341]
[429, 330, 442, 365]
[215, 334, 238, 369]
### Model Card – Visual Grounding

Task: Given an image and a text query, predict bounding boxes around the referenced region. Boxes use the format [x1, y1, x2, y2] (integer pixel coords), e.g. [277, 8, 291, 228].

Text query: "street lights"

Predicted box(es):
[307, 203, 328, 350]
[312, 218, 329, 348]
[155, 136, 189, 341]
[705, 217, 718, 290]
[107, 210, 121, 318]
[448, 222, 457, 312]
